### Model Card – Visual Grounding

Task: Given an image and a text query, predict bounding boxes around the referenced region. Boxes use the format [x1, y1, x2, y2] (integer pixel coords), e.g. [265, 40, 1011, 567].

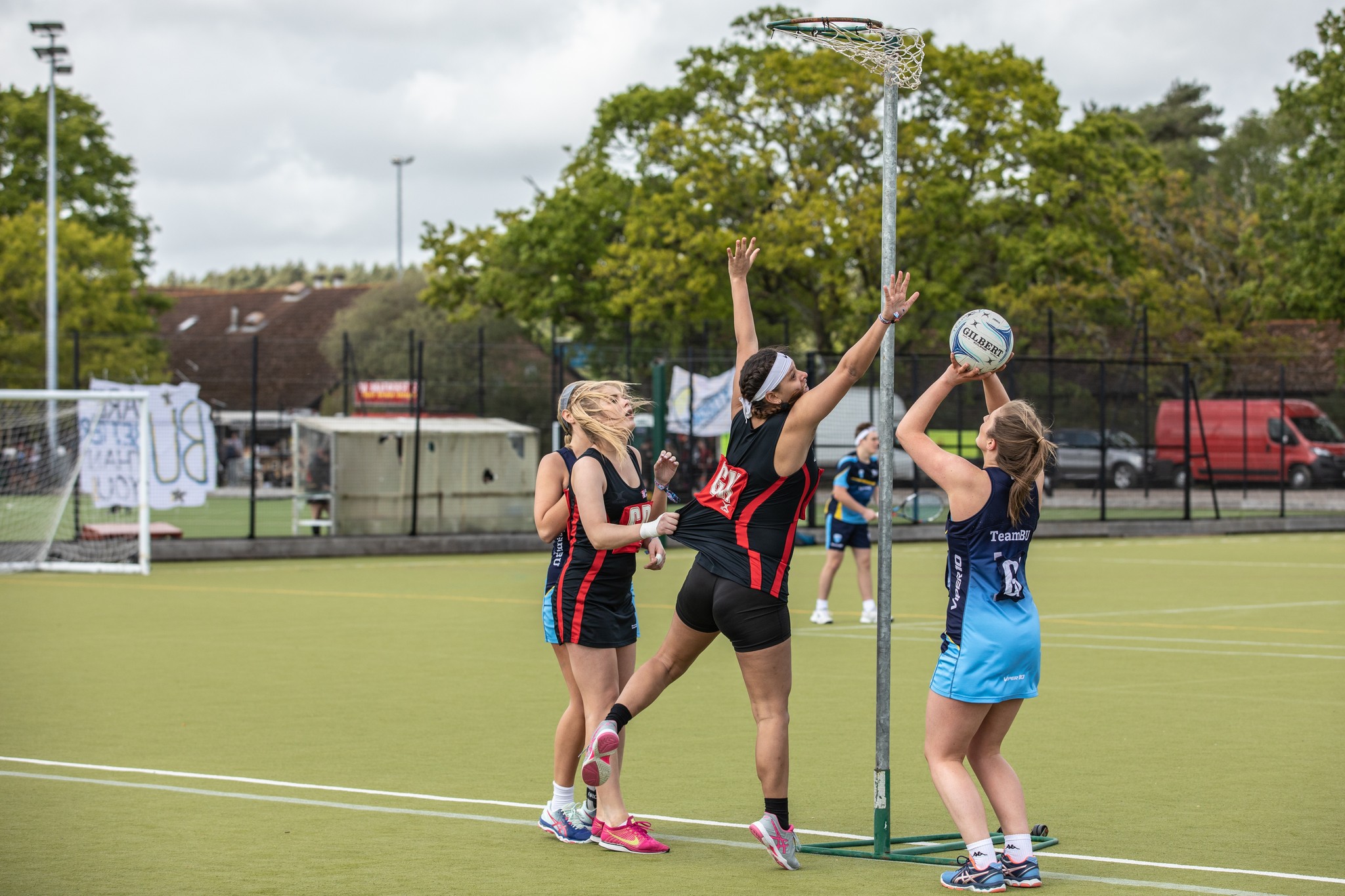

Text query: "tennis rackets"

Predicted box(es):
[874, 491, 945, 523]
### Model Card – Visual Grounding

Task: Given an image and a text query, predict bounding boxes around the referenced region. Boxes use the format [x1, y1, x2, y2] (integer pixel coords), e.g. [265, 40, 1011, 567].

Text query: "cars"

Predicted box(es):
[1047, 428, 1184, 491]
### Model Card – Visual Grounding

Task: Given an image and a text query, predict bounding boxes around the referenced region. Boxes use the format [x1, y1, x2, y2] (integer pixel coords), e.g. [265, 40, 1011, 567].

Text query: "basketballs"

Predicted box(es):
[947, 309, 1014, 375]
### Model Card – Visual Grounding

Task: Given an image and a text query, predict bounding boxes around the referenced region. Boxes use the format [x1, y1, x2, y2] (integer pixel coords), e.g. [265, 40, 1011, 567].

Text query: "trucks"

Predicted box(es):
[815, 385, 933, 486]
[1154, 396, 1345, 492]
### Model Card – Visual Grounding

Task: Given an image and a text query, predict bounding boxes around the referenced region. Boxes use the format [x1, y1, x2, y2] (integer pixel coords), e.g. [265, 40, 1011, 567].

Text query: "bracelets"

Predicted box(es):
[654, 478, 680, 503]
[644, 537, 661, 555]
[878, 308, 905, 324]
[640, 512, 666, 539]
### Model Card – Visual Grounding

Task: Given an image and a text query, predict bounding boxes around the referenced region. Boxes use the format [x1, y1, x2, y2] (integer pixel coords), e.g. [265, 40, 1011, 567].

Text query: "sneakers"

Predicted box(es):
[809, 606, 834, 625]
[940, 853, 1006, 892]
[861, 607, 894, 623]
[749, 811, 802, 870]
[577, 720, 620, 787]
[538, 801, 597, 843]
[590, 815, 671, 854]
[997, 846, 1042, 887]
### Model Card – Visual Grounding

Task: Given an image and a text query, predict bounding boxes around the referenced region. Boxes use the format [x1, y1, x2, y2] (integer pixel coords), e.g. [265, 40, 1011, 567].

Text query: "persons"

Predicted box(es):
[808, 422, 894, 626]
[222, 431, 331, 537]
[533, 382, 598, 845]
[551, 380, 680, 853]
[581, 233, 919, 871]
[896, 353, 1057, 894]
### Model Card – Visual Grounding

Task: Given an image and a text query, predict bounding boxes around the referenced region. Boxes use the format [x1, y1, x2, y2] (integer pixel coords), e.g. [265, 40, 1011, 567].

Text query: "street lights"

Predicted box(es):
[28, 17, 74, 458]
[390, 154, 417, 282]
